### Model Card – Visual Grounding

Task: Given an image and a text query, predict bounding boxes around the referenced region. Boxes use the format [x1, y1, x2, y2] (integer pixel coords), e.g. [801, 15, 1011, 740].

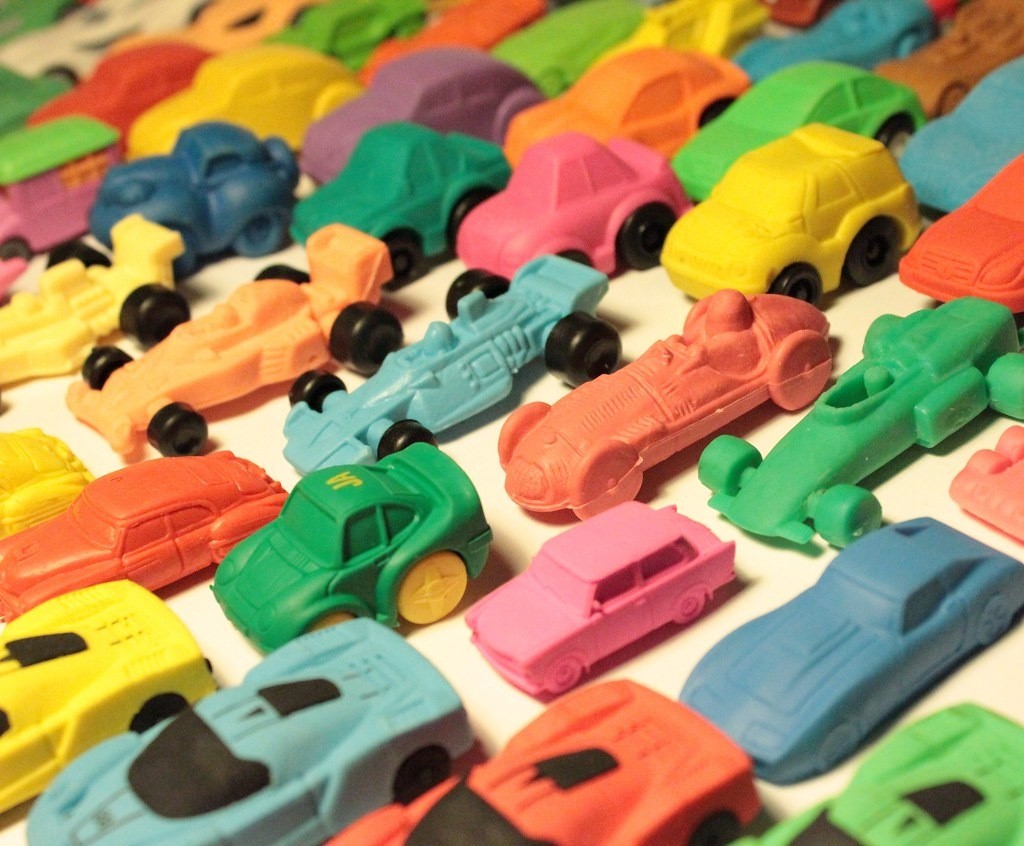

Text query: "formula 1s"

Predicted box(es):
[0, 212, 191, 385]
[62, 218, 404, 461]
[494, 288, 837, 519]
[281, 252, 625, 477]
[695, 295, 1024, 551]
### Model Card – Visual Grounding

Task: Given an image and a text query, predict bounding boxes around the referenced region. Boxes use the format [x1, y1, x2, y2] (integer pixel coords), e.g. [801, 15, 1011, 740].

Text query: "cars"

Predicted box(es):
[715, 702, 1022, 846]
[674, 515, 1023, 787]
[0, 578, 223, 816]
[208, 439, 494, 657]
[948, 422, 1024, 542]
[462, 500, 738, 696]
[23, 614, 475, 846]
[0, 427, 97, 542]
[324, 678, 764, 846]
[0, 450, 291, 625]
[0, 0, 1024, 314]
